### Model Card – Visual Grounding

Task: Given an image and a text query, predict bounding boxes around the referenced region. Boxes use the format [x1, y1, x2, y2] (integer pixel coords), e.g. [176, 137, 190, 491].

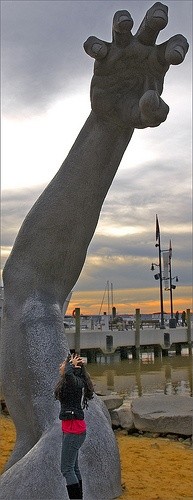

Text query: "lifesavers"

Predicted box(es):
[72, 309, 76, 317]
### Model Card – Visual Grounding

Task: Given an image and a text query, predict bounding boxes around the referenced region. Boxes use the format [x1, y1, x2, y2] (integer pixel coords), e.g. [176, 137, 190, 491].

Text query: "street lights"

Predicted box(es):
[150, 263, 165, 329]
[163, 276, 178, 328]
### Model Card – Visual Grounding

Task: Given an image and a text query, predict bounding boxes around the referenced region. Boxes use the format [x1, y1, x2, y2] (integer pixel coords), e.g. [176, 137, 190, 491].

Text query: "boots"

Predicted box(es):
[66, 479, 83, 500]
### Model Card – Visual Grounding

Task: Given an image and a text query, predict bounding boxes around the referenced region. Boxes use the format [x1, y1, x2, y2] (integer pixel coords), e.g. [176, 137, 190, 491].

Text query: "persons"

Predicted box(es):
[0, 1, 193, 500]
[54, 350, 95, 500]
[100, 312, 111, 331]
[175, 310, 180, 327]
[181, 311, 187, 326]
